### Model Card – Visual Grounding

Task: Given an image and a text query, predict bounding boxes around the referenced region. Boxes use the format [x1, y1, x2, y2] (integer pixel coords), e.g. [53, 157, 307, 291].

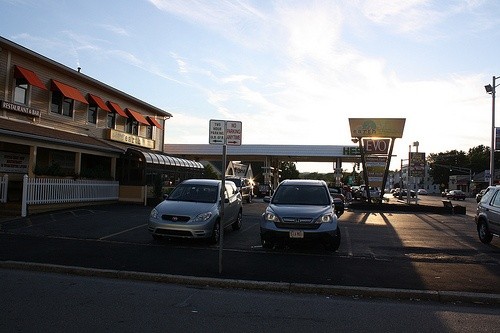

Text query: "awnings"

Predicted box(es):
[17, 66, 163, 130]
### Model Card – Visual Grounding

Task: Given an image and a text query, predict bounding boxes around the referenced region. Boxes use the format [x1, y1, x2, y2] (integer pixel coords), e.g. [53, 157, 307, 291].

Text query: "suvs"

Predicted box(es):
[473, 185, 500, 245]
[259, 178, 342, 252]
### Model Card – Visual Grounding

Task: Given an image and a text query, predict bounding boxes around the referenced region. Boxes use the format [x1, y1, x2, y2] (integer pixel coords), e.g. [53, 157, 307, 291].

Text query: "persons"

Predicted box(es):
[346, 186, 352, 202]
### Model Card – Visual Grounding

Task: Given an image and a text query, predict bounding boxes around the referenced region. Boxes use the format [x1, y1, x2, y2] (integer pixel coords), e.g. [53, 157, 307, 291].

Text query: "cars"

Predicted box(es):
[147, 179, 244, 246]
[225, 175, 488, 216]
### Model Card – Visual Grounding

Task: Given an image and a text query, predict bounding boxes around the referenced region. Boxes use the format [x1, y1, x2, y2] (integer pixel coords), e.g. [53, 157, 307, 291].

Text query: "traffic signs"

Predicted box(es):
[209, 119, 242, 146]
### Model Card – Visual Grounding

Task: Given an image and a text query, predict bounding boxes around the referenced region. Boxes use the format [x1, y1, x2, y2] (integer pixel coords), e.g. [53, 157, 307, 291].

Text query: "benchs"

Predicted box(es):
[442, 200, 465, 216]
